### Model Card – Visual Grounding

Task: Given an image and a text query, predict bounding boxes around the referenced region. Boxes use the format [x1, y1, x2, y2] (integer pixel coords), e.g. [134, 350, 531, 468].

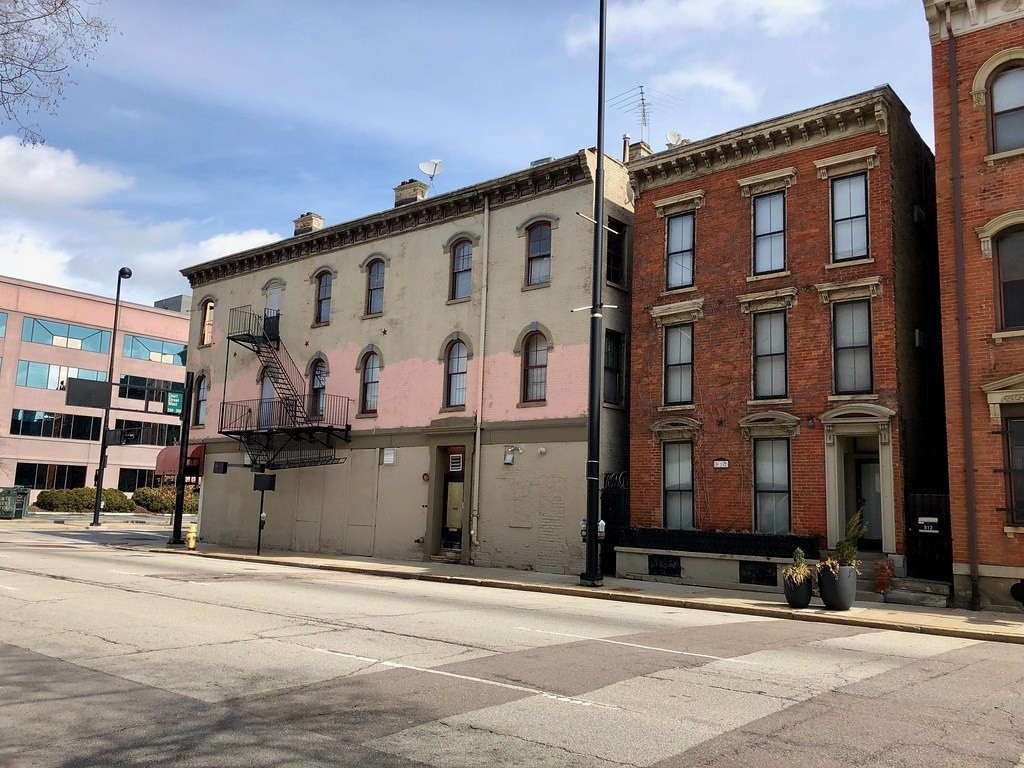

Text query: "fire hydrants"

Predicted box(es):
[185, 521, 199, 550]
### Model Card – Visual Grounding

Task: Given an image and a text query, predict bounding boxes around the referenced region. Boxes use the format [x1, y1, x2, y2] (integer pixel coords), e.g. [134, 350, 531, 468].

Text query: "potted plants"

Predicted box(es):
[781, 548, 814, 609]
[816, 506, 869, 609]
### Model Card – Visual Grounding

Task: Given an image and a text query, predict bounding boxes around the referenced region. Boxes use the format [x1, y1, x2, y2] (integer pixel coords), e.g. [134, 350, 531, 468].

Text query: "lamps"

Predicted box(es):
[805, 415, 821, 428]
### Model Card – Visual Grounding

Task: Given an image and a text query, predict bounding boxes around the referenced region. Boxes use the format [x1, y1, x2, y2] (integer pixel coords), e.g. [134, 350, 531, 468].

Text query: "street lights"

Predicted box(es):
[93, 268, 132, 525]
